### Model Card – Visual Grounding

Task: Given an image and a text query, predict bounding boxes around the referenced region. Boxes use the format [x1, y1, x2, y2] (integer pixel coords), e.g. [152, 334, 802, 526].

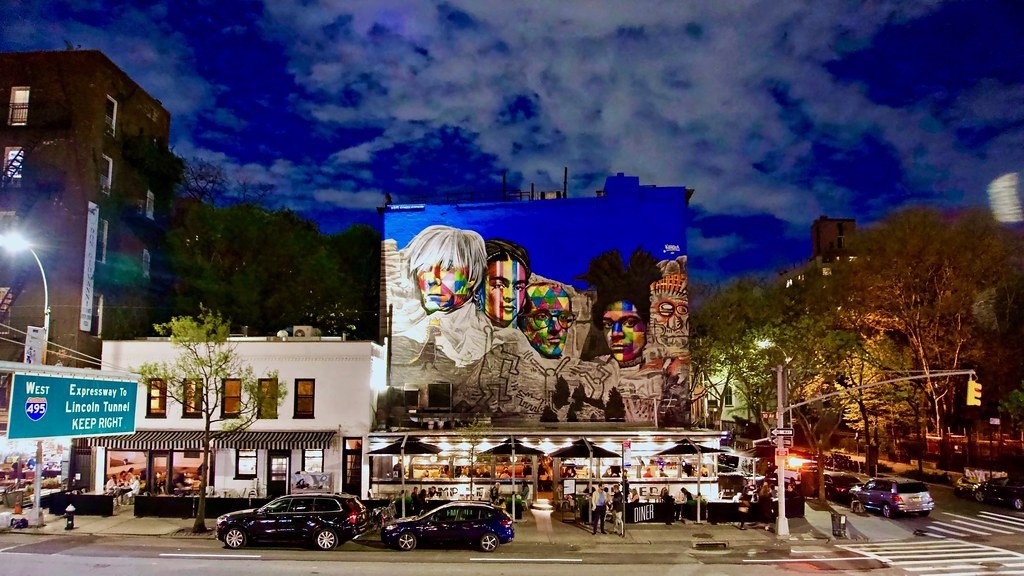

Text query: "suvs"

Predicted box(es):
[213, 491, 370, 552]
[852, 477, 935, 519]
[971, 476, 1024, 510]
[379, 499, 516, 553]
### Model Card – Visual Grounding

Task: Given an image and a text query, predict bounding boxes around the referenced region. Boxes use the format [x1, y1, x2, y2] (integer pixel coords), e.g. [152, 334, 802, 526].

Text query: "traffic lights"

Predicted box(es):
[967, 381, 982, 406]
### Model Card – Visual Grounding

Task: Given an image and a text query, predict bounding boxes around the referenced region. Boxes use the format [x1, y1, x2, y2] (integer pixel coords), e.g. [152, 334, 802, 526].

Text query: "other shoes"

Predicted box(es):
[601, 531, 607, 534]
[592, 532, 596, 535]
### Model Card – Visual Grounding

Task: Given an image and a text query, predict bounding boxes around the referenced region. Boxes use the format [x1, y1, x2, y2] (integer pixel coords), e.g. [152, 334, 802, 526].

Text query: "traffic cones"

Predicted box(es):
[14, 495, 23, 514]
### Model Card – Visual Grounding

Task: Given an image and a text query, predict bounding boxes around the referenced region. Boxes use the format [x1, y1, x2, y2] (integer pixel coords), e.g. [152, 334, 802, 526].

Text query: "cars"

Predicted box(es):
[823, 472, 866, 505]
[720, 431, 736, 445]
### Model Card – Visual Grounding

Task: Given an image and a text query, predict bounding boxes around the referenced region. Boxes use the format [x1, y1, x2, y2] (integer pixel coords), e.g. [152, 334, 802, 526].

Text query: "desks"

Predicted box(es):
[101, 488, 133, 506]
[173, 487, 192, 496]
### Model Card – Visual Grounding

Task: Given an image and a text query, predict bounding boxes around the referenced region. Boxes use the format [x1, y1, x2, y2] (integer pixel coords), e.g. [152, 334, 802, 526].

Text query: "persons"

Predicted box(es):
[560, 458, 709, 524]
[592, 481, 607, 535]
[611, 485, 623, 535]
[12, 456, 35, 483]
[733, 477, 802, 533]
[849, 490, 859, 514]
[393, 455, 550, 518]
[105, 467, 203, 507]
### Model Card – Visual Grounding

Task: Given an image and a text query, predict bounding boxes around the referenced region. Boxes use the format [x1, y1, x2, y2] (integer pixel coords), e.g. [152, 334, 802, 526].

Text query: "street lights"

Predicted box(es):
[755, 340, 794, 447]
[1, 230, 51, 364]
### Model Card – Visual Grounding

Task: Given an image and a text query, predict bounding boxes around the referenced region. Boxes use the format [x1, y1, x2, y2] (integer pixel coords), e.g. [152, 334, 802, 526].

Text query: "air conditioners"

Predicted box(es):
[293, 326, 313, 337]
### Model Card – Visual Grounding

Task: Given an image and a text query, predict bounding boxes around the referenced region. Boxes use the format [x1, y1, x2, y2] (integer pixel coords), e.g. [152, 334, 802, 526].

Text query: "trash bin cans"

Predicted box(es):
[832, 514, 846, 536]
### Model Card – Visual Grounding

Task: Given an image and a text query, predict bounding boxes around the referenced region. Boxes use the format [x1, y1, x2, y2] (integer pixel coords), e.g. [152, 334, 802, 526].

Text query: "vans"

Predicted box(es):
[773, 456, 819, 485]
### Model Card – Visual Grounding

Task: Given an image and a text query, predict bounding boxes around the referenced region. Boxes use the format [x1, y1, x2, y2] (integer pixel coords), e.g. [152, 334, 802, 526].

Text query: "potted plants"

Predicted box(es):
[687, 500, 707, 522]
[505, 498, 522, 519]
[395, 497, 412, 517]
[577, 496, 598, 522]
